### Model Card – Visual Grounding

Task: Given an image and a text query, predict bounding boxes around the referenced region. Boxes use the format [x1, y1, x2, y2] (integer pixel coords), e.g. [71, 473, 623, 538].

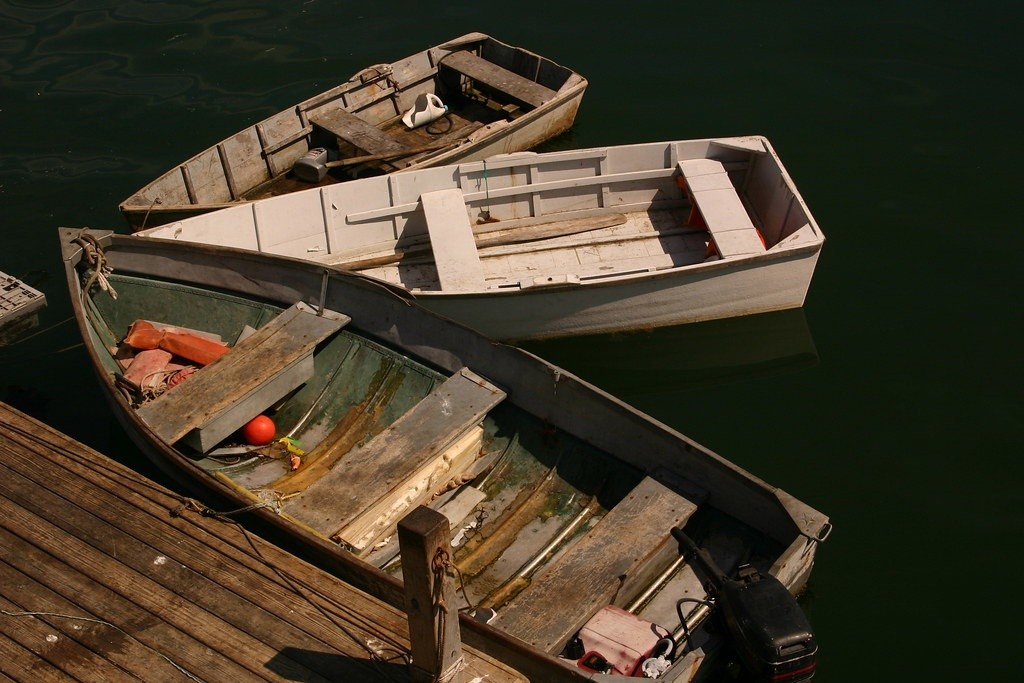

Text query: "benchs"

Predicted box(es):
[678, 157, 767, 263]
[280, 366, 511, 540]
[309, 107, 428, 170]
[421, 187, 486, 294]
[440, 50, 557, 109]
[486, 473, 698, 661]
[133, 301, 352, 447]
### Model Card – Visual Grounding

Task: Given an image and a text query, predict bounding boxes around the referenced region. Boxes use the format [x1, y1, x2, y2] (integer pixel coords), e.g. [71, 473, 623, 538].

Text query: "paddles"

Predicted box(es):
[323, 119, 485, 169]
[328, 212, 627, 271]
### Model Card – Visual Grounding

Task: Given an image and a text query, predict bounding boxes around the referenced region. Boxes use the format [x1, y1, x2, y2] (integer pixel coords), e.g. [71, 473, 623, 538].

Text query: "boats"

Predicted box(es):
[56, 210, 834, 683]
[116, 31, 591, 272]
[135, 133, 826, 352]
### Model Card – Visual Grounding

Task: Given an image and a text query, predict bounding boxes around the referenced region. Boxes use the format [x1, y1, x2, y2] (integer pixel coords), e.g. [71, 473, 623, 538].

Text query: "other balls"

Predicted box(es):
[242, 414, 277, 447]
